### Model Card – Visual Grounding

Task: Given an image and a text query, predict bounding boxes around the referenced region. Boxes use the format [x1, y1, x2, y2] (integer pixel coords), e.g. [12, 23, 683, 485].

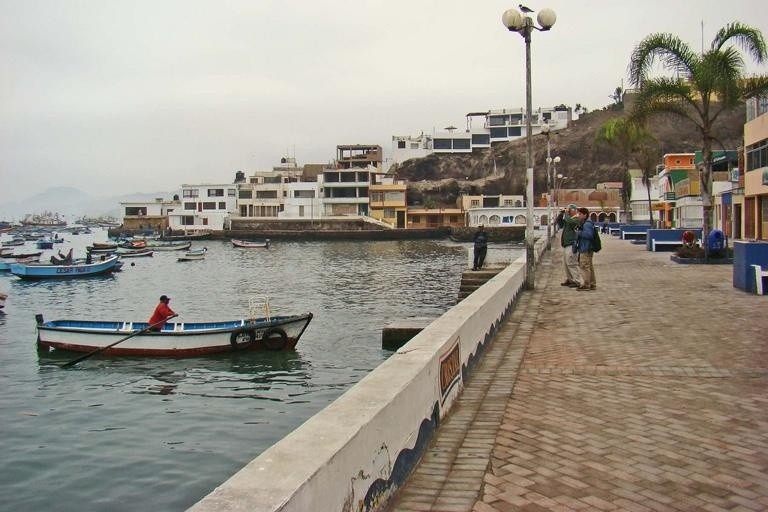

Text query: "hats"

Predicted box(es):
[567, 204, 577, 210]
[478, 223, 484, 227]
[160, 295, 171, 301]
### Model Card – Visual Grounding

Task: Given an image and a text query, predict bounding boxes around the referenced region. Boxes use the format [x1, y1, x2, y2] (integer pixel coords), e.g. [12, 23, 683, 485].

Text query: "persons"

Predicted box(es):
[471, 222, 488, 271]
[556, 204, 584, 288]
[148, 294, 179, 332]
[573, 207, 601, 290]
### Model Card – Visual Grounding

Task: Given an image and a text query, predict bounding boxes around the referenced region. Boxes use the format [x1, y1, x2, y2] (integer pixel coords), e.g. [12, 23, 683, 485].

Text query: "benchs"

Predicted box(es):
[599, 223, 608, 234]
[646, 228, 704, 252]
[734, 241, 768, 295]
[620, 226, 652, 239]
[608, 222, 634, 235]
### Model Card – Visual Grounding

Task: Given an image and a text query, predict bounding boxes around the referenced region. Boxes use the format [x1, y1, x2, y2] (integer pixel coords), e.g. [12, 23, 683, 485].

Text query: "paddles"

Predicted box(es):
[61, 314, 179, 369]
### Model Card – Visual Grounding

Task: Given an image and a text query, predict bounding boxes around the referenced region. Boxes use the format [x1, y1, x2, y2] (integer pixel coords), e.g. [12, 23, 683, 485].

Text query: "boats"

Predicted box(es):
[0, 210, 271, 315]
[36, 313, 313, 355]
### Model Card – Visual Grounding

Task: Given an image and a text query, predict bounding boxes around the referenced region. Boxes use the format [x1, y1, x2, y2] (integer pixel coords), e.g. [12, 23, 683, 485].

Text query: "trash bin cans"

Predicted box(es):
[683, 231, 696, 249]
[704, 230, 728, 264]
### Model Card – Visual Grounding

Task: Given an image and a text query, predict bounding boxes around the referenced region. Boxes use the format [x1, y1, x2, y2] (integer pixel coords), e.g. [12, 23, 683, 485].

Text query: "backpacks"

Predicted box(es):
[586, 220, 602, 253]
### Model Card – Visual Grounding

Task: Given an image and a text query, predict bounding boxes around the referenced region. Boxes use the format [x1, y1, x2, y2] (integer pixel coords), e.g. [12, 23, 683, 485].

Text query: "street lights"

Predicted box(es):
[502, 9, 568, 289]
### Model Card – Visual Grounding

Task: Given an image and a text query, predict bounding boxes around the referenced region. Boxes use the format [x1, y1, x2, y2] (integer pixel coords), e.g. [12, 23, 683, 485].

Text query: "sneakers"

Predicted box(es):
[569, 281, 581, 288]
[471, 267, 481, 271]
[576, 285, 590, 291]
[590, 284, 597, 290]
[560, 279, 572, 286]
